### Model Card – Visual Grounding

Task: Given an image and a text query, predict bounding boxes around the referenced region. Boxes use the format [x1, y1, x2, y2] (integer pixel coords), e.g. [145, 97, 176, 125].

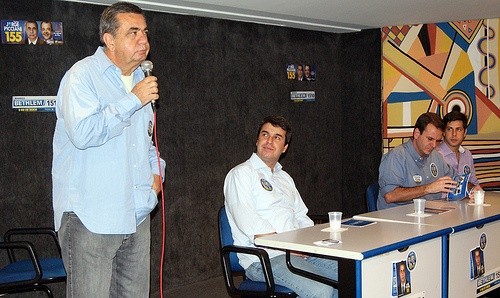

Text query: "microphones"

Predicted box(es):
[141, 60, 158, 109]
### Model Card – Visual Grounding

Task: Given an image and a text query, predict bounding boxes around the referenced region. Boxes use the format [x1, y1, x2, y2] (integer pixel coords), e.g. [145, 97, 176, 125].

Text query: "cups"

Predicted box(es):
[328, 211, 343, 230]
[413, 198, 426, 214]
[473, 191, 485, 204]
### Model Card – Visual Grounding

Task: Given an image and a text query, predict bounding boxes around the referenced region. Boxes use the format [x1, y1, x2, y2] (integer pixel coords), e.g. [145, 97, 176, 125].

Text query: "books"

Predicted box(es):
[341, 218, 377, 228]
[436, 174, 471, 202]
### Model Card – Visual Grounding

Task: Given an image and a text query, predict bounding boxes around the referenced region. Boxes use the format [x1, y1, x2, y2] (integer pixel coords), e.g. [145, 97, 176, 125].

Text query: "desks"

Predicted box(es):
[256, 190, 500, 298]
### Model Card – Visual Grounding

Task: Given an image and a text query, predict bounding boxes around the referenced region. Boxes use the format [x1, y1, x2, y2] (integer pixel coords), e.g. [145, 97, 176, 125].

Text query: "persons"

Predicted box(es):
[40, 20, 63, 44]
[294, 64, 307, 81]
[52, 1, 166, 298]
[436, 112, 484, 202]
[19, 20, 47, 45]
[481, 235, 485, 248]
[474, 248, 486, 278]
[223, 116, 338, 298]
[398, 261, 411, 295]
[408, 254, 416, 270]
[304, 64, 316, 80]
[377, 112, 457, 210]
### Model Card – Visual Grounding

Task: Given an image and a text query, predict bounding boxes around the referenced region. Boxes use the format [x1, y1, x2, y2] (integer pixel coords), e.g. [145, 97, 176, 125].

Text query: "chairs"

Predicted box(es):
[366, 183, 379, 212]
[0, 227, 68, 298]
[218, 205, 299, 298]
